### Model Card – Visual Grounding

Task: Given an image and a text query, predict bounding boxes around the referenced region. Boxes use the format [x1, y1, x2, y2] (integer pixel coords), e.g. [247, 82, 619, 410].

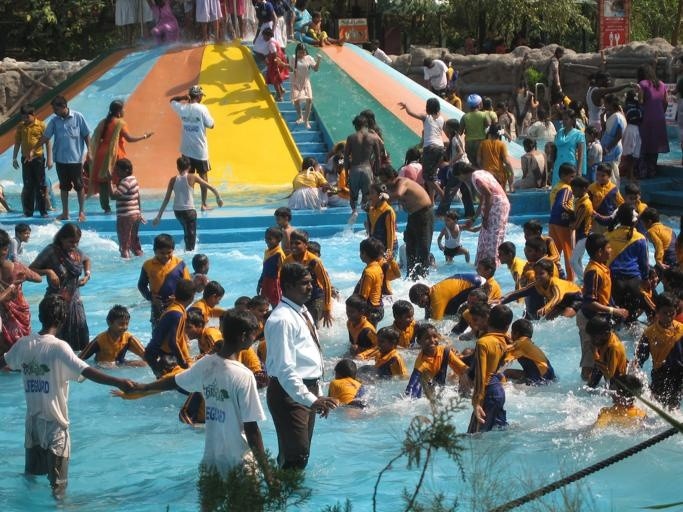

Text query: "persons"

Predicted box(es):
[0, 0, 683, 512]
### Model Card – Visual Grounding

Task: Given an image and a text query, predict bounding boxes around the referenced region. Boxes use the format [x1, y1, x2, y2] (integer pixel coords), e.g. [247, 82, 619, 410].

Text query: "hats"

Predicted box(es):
[189, 85, 206, 97]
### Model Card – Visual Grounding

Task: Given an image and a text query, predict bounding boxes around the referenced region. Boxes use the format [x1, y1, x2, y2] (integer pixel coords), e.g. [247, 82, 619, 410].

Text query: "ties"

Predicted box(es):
[302, 311, 320, 353]
